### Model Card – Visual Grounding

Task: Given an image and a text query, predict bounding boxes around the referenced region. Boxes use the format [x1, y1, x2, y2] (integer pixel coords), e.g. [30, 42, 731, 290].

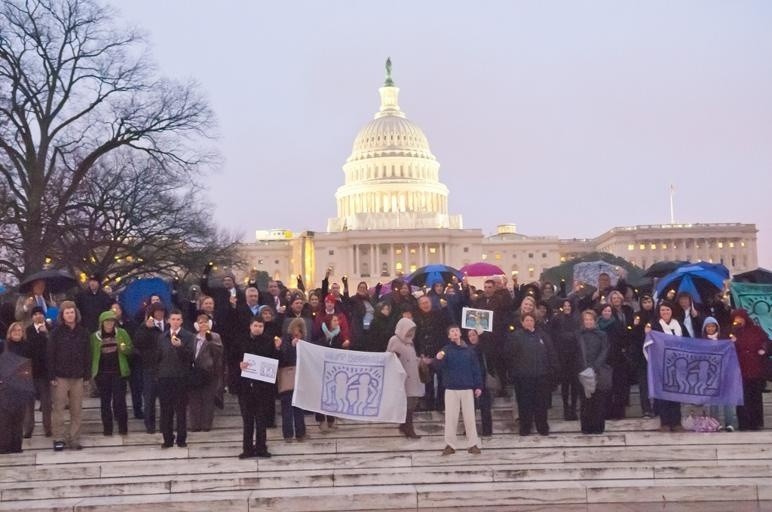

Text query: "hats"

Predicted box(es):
[324, 294, 336, 303]
[255, 304, 267, 316]
[290, 293, 302, 303]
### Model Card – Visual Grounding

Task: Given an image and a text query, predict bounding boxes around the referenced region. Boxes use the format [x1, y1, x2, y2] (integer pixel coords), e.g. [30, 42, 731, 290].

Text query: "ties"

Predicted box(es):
[157, 322, 161, 329]
[275, 298, 278, 306]
[172, 330, 176, 336]
[38, 296, 43, 308]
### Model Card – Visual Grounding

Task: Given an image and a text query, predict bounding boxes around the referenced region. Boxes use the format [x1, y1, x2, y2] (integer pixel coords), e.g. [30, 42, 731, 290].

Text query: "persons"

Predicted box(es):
[728, 308, 769, 430]
[0, 261, 728, 459]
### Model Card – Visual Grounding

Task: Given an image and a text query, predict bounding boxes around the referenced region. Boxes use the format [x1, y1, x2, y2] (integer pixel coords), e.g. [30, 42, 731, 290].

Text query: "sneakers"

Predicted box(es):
[726, 425, 734, 432]
[716, 425, 723, 432]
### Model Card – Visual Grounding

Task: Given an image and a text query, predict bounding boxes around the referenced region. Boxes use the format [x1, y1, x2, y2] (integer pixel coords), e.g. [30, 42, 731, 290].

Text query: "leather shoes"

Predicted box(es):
[255, 451, 271, 457]
[239, 449, 254, 459]
[468, 445, 480, 454]
[443, 445, 455, 455]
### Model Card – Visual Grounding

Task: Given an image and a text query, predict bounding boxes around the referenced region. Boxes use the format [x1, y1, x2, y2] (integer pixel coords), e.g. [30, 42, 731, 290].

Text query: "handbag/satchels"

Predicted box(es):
[484, 373, 503, 394]
[418, 360, 431, 384]
